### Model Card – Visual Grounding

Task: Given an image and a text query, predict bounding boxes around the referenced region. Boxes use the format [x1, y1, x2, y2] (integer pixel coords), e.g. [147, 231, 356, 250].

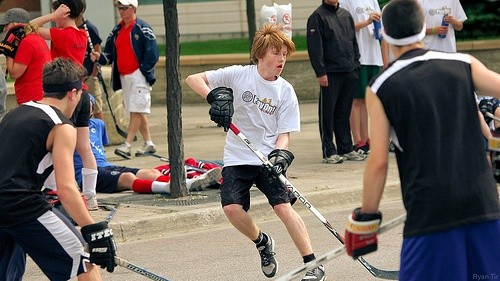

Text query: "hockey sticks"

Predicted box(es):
[159, 158, 206, 173]
[273, 214, 407, 281]
[83, 243, 171, 281]
[230, 122, 401, 280]
[83, 23, 138, 141]
[97, 201, 121, 224]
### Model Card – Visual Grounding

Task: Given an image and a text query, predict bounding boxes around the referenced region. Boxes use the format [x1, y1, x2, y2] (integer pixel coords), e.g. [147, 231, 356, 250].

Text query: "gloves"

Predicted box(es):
[0, 26, 26, 59]
[260, 149, 295, 192]
[207, 87, 234, 132]
[345, 208, 383, 257]
[478, 97, 500, 125]
[80, 221, 118, 273]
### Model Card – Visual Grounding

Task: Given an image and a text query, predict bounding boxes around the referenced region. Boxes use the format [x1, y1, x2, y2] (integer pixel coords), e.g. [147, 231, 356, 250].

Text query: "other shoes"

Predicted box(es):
[86, 194, 99, 210]
[353, 138, 370, 154]
[137, 142, 157, 154]
[187, 167, 221, 192]
[115, 144, 132, 159]
[389, 141, 396, 152]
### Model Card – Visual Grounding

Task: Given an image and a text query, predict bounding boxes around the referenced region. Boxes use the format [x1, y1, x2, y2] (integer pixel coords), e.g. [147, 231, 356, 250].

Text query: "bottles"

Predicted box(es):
[439, 14, 450, 38]
[373, 16, 383, 40]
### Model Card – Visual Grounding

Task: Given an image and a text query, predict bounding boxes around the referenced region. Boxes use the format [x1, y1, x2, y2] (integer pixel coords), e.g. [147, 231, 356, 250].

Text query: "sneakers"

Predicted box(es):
[256, 232, 278, 277]
[322, 155, 343, 164]
[340, 151, 366, 161]
[301, 264, 327, 281]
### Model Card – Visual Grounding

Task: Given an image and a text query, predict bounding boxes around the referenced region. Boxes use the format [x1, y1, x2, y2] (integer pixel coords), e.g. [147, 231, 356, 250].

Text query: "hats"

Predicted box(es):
[118, 0, 138, 8]
[0, 8, 29, 24]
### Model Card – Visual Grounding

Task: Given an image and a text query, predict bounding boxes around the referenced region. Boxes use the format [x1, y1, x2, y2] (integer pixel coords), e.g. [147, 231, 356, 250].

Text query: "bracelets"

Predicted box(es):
[432, 26, 434, 35]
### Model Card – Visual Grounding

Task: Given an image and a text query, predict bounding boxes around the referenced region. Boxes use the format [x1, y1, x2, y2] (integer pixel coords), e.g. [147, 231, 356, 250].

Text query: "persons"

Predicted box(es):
[473, 92, 500, 177]
[74, 92, 222, 195]
[0, 0, 103, 211]
[306, 0, 390, 163]
[344, 0, 500, 281]
[419, 0, 467, 52]
[91, 0, 159, 159]
[0, 58, 118, 281]
[185, 22, 327, 281]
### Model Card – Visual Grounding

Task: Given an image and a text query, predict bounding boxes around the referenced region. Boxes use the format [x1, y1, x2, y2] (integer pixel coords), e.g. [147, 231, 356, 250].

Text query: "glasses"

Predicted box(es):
[118, 6, 132, 10]
